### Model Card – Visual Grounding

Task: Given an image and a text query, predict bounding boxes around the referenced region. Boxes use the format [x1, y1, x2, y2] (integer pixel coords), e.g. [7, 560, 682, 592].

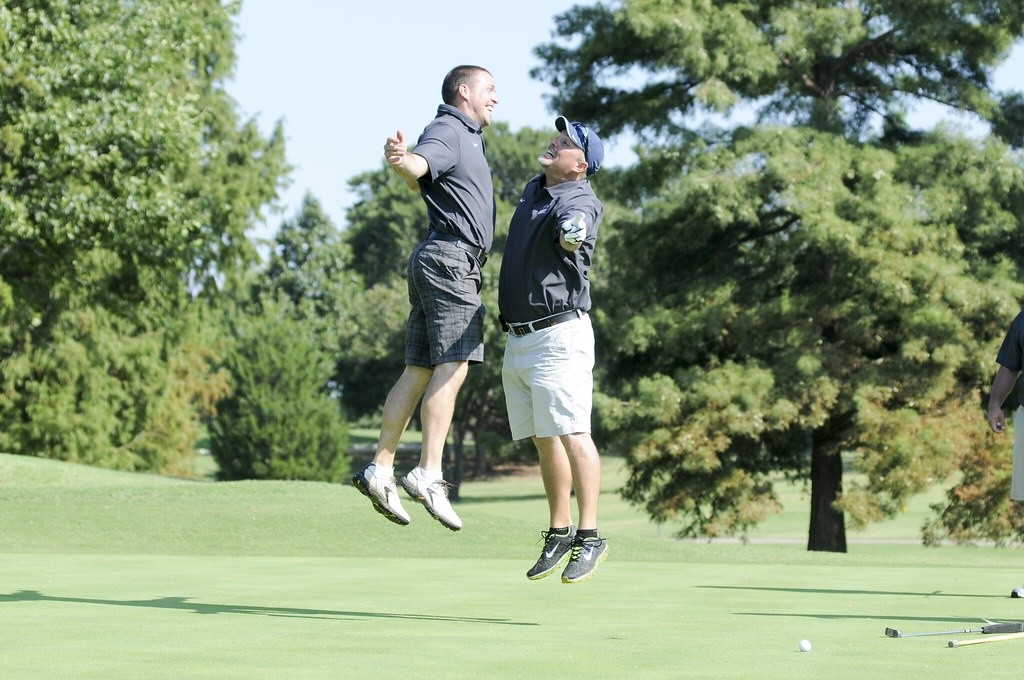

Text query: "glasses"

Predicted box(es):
[571, 122, 589, 161]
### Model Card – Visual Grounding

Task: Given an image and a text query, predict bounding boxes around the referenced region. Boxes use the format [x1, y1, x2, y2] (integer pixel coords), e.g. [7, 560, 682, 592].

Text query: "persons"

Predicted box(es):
[988, 309, 1024, 597]
[498, 116, 608, 583]
[355, 66, 498, 530]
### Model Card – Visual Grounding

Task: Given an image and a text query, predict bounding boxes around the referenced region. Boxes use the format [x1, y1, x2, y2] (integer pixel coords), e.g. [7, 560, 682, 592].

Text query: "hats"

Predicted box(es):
[556, 113, 604, 177]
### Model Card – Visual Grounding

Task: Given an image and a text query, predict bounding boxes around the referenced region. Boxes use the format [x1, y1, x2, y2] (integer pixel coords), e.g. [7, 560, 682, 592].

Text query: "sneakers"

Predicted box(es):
[561, 532, 609, 583]
[352, 462, 410, 527]
[526, 526, 579, 580]
[400, 465, 462, 533]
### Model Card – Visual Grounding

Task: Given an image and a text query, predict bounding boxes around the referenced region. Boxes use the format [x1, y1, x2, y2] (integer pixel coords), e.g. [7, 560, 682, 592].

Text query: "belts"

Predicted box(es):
[428, 225, 488, 268]
[505, 308, 587, 336]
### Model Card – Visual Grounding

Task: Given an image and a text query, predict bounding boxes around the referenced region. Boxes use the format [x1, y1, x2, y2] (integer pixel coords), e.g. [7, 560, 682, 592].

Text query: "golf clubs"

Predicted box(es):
[884, 622, 1023, 638]
[948, 633, 1023, 647]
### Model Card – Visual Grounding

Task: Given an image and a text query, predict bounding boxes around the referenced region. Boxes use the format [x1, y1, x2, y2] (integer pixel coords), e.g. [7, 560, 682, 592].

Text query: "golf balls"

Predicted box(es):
[799, 640, 812, 652]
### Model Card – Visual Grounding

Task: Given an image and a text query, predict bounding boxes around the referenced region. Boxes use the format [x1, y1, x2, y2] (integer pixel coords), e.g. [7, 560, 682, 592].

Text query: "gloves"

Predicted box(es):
[562, 214, 588, 245]
